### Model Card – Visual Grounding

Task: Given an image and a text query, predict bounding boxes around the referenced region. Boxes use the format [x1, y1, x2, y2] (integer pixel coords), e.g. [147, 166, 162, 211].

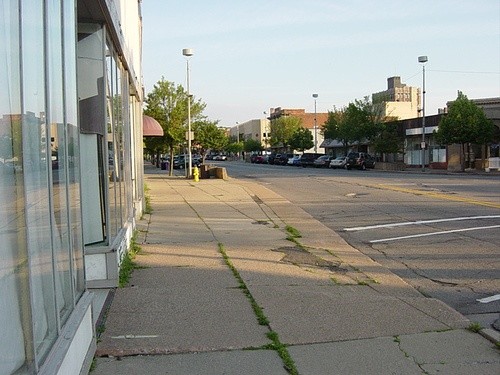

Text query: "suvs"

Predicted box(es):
[346, 151, 376, 171]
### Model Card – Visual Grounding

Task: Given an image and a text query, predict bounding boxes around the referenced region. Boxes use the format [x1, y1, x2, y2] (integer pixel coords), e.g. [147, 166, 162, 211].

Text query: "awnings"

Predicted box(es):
[143, 114, 163, 136]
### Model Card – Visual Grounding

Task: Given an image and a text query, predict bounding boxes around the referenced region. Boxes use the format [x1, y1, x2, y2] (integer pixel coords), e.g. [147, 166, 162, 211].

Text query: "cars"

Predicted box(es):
[312, 154, 337, 168]
[329, 156, 349, 169]
[249, 150, 327, 168]
[151, 153, 228, 170]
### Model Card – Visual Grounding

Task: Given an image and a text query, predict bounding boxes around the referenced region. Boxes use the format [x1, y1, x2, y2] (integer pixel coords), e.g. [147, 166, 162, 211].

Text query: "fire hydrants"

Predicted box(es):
[193, 166, 200, 182]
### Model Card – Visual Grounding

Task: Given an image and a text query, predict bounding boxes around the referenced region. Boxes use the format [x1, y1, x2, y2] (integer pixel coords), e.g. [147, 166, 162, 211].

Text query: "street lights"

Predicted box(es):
[312, 93, 318, 153]
[264, 111, 268, 154]
[236, 121, 240, 160]
[182, 48, 195, 179]
[418, 55, 429, 173]
